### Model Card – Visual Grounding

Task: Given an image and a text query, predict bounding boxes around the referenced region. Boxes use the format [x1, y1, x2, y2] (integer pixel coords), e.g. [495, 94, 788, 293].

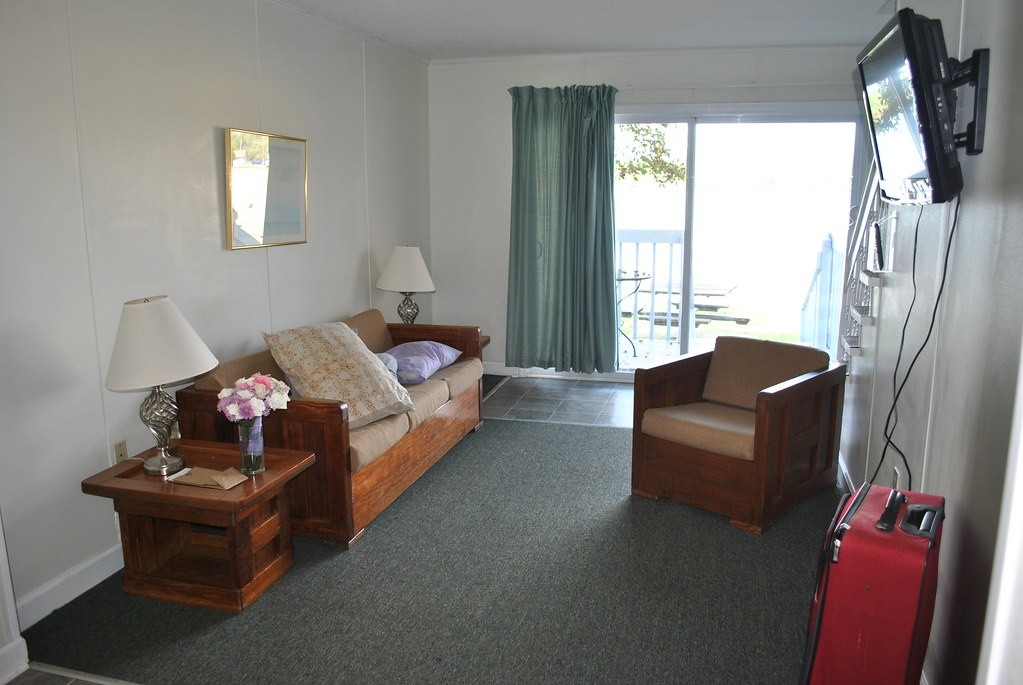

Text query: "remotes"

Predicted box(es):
[873, 223, 884, 271]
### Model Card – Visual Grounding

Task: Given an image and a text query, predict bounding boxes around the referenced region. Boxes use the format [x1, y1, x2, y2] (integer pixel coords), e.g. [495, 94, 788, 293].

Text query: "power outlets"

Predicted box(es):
[113, 440, 129, 460]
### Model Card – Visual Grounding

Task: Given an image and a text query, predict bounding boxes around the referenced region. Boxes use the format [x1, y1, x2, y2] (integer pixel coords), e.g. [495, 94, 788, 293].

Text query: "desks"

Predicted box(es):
[641, 283, 738, 327]
[615, 270, 652, 358]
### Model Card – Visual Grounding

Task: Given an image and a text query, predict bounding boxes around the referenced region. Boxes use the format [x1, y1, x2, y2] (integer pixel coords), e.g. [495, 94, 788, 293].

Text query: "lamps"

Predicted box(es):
[376, 245, 436, 320]
[106, 293, 219, 473]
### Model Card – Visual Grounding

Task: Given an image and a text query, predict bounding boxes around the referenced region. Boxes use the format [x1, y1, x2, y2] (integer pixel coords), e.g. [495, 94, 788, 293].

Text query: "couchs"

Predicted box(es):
[626, 335, 847, 536]
[172, 324, 484, 551]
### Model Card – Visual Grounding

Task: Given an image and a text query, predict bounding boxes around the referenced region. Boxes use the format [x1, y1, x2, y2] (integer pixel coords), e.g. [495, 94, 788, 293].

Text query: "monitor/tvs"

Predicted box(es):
[857, 8, 963, 207]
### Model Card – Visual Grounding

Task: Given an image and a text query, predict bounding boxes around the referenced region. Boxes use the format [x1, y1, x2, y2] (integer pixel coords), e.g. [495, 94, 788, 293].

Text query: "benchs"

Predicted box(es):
[639, 300, 751, 325]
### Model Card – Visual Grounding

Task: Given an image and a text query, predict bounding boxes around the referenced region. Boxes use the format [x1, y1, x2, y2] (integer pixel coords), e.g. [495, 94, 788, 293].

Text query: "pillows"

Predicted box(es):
[384, 340, 463, 384]
[259, 320, 414, 427]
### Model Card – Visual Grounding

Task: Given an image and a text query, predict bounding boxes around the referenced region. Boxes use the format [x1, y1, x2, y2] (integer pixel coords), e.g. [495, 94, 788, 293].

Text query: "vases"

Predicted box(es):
[237, 417, 265, 475]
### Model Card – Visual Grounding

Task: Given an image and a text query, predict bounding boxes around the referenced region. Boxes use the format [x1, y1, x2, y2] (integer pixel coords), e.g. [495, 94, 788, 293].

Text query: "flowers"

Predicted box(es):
[213, 371, 290, 420]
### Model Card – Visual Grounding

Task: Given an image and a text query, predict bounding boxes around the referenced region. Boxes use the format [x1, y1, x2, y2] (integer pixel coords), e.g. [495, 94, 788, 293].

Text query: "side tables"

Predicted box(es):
[76, 440, 316, 612]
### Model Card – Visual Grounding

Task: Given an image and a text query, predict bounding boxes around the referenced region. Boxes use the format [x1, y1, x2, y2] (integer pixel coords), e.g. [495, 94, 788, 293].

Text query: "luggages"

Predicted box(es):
[796, 481, 946, 685]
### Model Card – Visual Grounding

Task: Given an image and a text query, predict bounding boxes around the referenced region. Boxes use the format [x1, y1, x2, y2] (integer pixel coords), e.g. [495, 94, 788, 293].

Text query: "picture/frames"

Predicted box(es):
[225, 126, 307, 250]
